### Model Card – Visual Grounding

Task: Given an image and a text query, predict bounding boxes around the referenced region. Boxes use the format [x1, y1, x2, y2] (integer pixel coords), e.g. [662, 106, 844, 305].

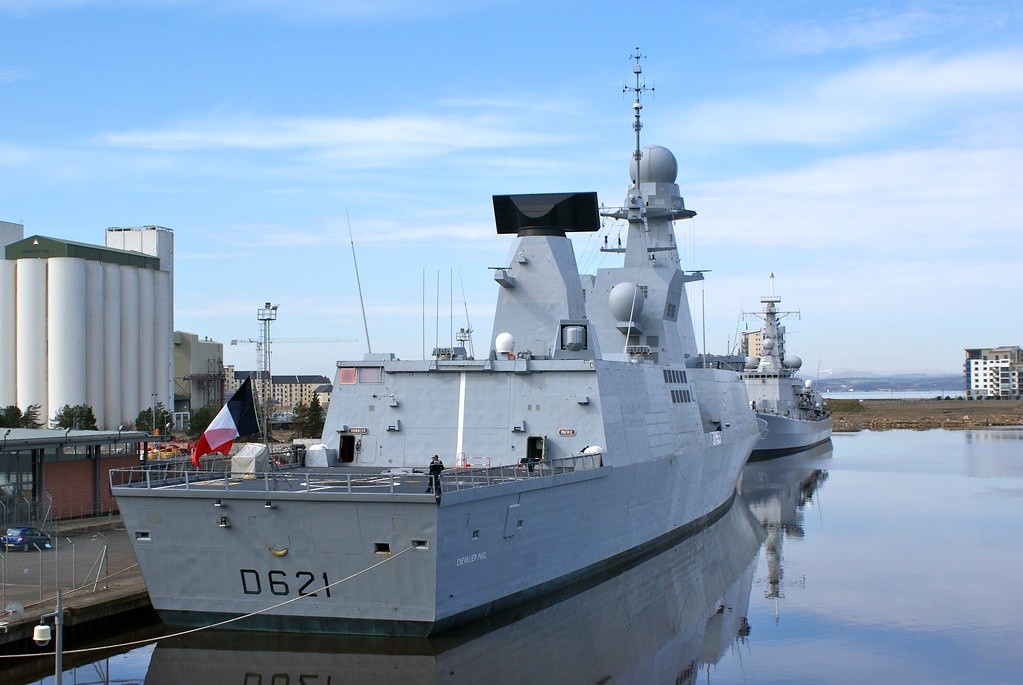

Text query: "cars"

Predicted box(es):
[0, 527, 52, 552]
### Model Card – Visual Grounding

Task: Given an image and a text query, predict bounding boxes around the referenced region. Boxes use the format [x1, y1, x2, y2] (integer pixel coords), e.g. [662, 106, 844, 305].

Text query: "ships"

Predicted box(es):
[731, 275, 835, 453]
[101, 44, 768, 639]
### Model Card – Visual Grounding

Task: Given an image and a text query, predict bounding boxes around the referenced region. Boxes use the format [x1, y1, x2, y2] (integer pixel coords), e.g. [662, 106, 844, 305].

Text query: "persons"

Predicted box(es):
[425, 453, 445, 495]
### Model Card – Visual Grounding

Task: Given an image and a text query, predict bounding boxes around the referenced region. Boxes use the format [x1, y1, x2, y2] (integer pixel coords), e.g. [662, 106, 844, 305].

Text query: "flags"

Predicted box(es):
[190, 375, 260, 469]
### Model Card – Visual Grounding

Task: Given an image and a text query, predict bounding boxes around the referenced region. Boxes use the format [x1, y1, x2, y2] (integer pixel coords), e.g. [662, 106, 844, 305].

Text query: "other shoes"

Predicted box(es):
[432, 491, 437, 494]
[425, 490, 430, 493]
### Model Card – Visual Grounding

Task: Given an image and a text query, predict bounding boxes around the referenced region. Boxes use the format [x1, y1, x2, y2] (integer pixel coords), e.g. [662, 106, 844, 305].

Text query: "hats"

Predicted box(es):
[431, 455, 438, 459]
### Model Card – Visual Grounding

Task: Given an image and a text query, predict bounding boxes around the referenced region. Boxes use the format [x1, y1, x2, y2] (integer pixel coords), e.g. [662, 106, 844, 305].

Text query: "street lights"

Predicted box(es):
[33, 589, 65, 685]
